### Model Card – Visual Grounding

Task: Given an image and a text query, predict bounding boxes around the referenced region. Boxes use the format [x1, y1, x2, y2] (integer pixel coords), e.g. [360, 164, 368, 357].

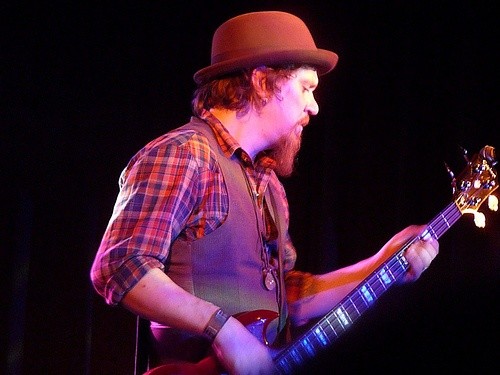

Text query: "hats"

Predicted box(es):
[193, 11, 339, 86]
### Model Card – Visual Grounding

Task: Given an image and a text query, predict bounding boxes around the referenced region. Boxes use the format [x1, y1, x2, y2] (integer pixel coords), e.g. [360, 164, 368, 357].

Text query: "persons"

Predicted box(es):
[89, 7, 443, 375]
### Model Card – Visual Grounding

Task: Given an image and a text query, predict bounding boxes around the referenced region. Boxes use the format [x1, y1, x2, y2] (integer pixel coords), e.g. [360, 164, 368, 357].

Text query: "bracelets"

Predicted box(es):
[197, 305, 235, 346]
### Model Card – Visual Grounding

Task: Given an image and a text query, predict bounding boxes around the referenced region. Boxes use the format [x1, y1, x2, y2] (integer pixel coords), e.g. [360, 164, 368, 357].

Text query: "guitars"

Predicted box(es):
[146, 143, 499, 374]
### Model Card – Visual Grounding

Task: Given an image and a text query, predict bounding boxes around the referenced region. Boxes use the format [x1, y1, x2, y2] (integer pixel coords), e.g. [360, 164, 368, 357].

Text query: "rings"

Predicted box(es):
[423, 267, 427, 270]
